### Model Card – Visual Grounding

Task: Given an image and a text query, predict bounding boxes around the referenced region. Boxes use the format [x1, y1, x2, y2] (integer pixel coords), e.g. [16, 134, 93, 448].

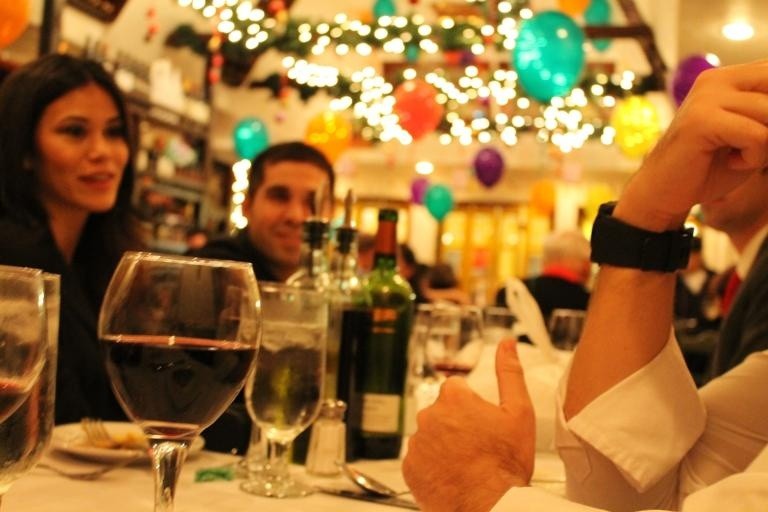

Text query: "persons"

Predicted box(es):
[355, 232, 449, 310]
[402, 60, 765, 511]
[136, 200, 233, 262]
[496, 226, 594, 349]
[150, 140, 337, 465]
[0, 52, 154, 429]
[677, 226, 722, 385]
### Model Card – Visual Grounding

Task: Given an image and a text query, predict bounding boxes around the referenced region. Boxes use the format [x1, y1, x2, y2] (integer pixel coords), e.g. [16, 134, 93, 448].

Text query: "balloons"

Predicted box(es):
[225, 1, 768, 224]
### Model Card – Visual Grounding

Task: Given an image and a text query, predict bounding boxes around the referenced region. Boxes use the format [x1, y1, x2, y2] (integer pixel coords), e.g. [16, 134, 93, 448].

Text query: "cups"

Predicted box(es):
[544, 309, 588, 352]
[0, 266, 60, 498]
[415, 301, 462, 376]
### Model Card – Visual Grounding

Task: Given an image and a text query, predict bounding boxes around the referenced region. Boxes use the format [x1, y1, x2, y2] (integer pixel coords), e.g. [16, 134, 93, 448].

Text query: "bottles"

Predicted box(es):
[284, 210, 414, 465]
[470, 309, 519, 407]
[305, 399, 346, 475]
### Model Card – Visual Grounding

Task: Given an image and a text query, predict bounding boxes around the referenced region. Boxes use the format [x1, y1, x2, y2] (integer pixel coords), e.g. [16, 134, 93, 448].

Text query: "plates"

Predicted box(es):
[45, 421, 206, 464]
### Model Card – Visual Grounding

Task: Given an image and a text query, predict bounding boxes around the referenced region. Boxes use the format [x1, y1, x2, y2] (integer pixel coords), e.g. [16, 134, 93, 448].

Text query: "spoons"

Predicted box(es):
[333, 460, 411, 498]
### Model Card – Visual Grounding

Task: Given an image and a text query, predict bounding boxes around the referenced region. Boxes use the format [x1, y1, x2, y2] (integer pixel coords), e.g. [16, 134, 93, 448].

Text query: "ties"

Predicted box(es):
[719, 270, 741, 318]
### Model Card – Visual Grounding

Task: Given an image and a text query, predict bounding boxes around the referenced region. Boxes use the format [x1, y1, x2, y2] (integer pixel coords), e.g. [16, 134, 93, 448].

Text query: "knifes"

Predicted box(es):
[313, 483, 420, 512]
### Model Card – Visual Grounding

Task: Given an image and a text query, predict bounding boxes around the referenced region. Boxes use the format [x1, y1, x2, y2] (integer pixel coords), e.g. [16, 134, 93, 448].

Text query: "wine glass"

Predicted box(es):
[99, 251, 329, 512]
